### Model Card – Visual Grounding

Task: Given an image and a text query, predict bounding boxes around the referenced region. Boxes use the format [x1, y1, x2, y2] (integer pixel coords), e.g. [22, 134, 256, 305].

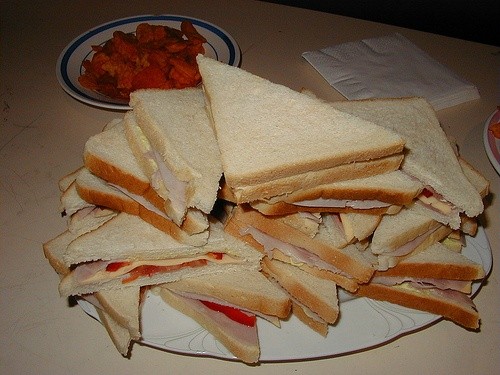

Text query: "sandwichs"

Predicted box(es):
[42, 53, 491, 365]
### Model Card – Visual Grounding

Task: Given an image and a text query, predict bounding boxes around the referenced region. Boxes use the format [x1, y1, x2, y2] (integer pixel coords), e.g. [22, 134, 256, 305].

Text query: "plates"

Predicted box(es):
[483, 105, 500, 176]
[73, 215, 493, 362]
[55, 14, 241, 110]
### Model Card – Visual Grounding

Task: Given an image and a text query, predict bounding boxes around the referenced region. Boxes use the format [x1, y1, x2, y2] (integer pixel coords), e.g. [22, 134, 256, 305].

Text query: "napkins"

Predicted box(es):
[301, 32, 481, 116]
